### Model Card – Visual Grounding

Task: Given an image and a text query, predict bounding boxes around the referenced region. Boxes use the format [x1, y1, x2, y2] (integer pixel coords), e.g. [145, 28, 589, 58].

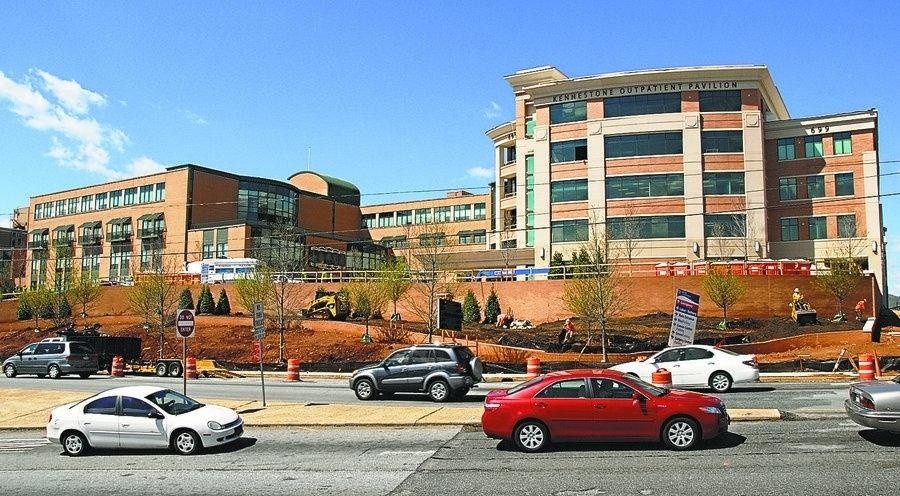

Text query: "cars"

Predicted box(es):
[843, 374, 899, 439]
[480, 367, 735, 454]
[47, 385, 244, 456]
[607, 344, 761, 393]
[271, 274, 289, 282]
[475, 270, 497, 280]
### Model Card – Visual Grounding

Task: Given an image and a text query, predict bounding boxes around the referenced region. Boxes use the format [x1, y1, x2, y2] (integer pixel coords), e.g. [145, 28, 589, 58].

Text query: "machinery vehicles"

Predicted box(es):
[298, 290, 351, 320]
[789, 288, 820, 325]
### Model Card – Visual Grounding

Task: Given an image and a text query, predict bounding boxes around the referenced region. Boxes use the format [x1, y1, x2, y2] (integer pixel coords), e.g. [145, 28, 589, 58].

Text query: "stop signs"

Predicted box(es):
[177, 307, 196, 338]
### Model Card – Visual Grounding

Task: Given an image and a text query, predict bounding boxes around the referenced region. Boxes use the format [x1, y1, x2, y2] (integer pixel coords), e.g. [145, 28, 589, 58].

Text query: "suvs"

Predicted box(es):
[348, 342, 484, 402]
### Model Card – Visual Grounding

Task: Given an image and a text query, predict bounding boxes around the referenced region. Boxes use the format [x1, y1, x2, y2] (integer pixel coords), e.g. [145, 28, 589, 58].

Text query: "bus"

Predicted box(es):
[201, 257, 268, 283]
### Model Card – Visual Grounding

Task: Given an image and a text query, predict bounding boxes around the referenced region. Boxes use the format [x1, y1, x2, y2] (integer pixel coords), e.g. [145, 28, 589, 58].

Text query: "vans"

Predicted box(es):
[1, 340, 100, 380]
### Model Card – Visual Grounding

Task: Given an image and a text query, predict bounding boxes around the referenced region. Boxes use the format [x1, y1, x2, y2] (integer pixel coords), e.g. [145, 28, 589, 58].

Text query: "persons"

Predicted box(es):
[495, 314, 506, 328]
[503, 316, 514, 329]
[793, 287, 803, 303]
[853, 299, 867, 320]
[563, 319, 575, 343]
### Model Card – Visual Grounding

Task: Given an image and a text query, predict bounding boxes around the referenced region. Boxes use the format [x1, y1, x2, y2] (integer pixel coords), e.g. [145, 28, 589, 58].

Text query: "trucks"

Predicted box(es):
[40, 334, 142, 372]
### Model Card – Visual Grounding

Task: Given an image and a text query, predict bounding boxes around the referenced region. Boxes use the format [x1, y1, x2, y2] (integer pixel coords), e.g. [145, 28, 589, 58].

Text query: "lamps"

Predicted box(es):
[768, 244, 773, 255]
[540, 248, 544, 258]
[693, 242, 700, 256]
[756, 242, 763, 251]
